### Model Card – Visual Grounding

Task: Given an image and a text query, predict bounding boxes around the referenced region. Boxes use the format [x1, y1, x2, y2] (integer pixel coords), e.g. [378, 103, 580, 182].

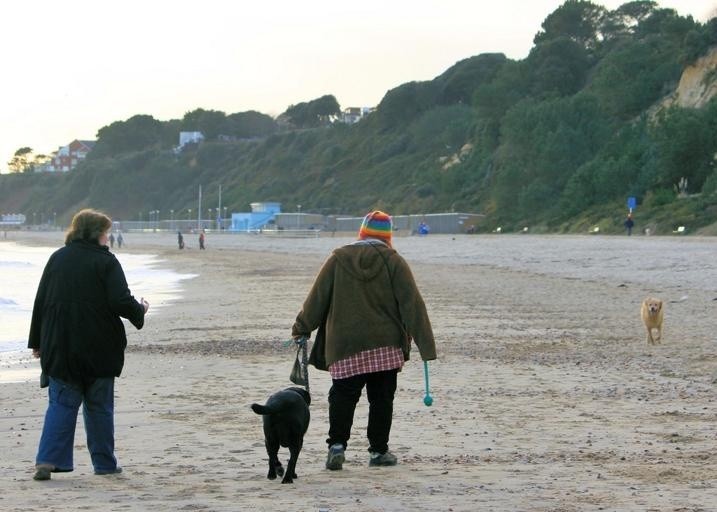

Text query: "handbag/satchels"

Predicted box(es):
[288, 343, 310, 387]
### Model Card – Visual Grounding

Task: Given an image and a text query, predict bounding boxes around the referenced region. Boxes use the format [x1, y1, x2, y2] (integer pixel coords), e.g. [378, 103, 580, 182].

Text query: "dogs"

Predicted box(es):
[250, 387, 311, 484]
[640, 297, 665, 347]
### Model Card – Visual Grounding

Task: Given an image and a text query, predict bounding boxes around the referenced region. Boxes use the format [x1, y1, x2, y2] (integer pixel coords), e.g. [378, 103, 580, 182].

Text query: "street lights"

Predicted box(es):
[139, 210, 160, 231]
[3, 213, 56, 232]
[170, 210, 174, 230]
[208, 207, 228, 230]
[188, 210, 192, 229]
[297, 205, 301, 228]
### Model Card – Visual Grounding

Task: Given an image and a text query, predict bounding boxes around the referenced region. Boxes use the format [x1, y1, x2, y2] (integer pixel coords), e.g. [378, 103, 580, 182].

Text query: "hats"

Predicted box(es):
[356, 210, 392, 248]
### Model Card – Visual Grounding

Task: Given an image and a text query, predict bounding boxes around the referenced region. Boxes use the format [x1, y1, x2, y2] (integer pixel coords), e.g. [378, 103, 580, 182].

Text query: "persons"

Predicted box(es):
[109, 233, 115, 248]
[117, 233, 123, 249]
[27, 208, 149, 480]
[199, 231, 205, 249]
[624, 214, 633, 236]
[177, 231, 184, 249]
[292, 209, 436, 469]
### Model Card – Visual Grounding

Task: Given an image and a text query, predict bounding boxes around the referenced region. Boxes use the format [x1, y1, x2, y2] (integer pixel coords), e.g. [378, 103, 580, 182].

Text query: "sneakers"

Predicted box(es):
[368, 450, 397, 467]
[33, 466, 52, 481]
[94, 467, 123, 476]
[325, 446, 344, 469]
